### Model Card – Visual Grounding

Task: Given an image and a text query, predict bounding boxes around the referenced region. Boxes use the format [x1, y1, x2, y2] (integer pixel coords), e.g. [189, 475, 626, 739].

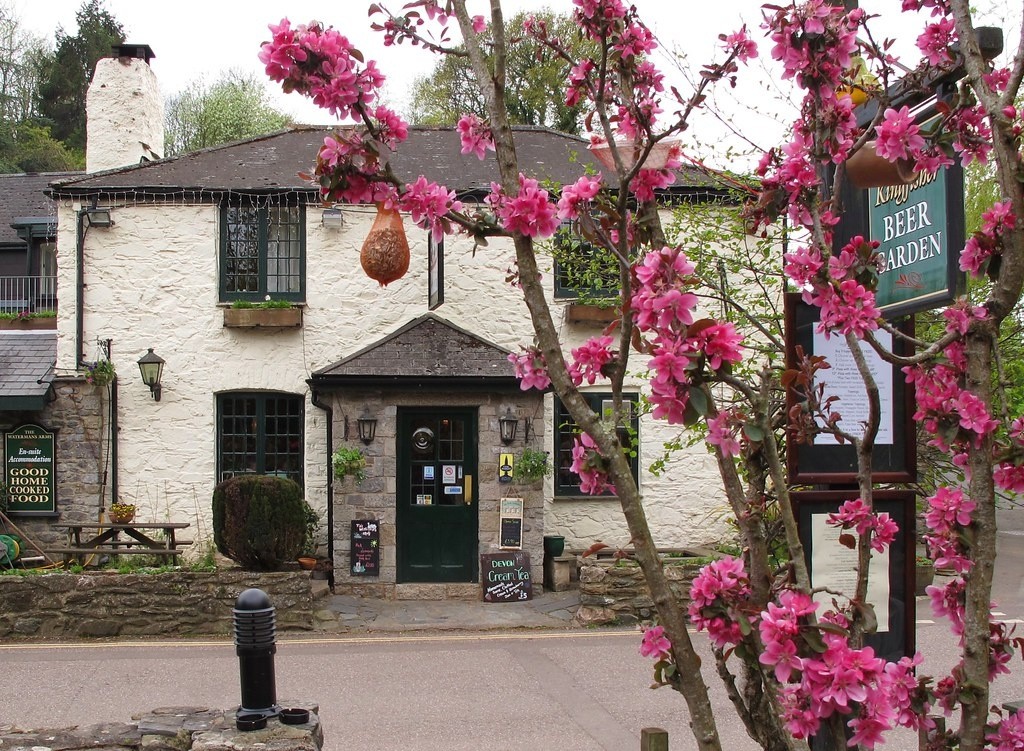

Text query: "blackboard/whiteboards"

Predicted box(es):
[499, 498, 523, 550]
[480, 551, 533, 602]
[351, 519, 379, 577]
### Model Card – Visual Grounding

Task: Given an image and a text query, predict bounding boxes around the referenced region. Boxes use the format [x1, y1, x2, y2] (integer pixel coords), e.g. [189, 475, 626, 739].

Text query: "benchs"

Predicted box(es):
[71, 541, 194, 546]
[44, 548, 184, 555]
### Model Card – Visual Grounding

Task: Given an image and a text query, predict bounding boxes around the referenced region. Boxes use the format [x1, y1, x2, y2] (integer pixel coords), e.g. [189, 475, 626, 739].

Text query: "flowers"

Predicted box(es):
[232, 294, 291, 309]
[0, 311, 57, 323]
[108, 502, 138, 523]
[916, 552, 933, 567]
[512, 447, 553, 482]
[331, 446, 369, 487]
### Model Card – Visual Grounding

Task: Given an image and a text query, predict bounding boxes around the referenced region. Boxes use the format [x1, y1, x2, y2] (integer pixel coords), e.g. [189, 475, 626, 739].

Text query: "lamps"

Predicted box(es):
[321, 209, 344, 228]
[85, 209, 117, 229]
[136, 348, 166, 402]
[498, 408, 519, 445]
[357, 407, 378, 446]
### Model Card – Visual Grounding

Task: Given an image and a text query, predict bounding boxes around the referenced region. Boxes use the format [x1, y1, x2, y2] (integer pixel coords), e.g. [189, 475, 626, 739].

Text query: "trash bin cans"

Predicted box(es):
[931, 556, 969, 611]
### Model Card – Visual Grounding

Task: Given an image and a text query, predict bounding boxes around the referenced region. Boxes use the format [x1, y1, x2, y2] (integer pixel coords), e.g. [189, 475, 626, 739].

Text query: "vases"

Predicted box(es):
[298, 557, 316, 571]
[223, 308, 302, 329]
[915, 565, 936, 596]
[0, 318, 57, 330]
[109, 512, 134, 523]
[544, 535, 566, 556]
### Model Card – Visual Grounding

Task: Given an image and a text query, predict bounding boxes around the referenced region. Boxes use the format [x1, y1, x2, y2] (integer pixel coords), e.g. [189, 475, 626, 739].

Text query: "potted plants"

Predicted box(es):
[565, 296, 623, 324]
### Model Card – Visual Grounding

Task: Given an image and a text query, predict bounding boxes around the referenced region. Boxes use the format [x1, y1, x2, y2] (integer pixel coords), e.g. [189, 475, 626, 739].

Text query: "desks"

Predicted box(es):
[51, 522, 190, 570]
